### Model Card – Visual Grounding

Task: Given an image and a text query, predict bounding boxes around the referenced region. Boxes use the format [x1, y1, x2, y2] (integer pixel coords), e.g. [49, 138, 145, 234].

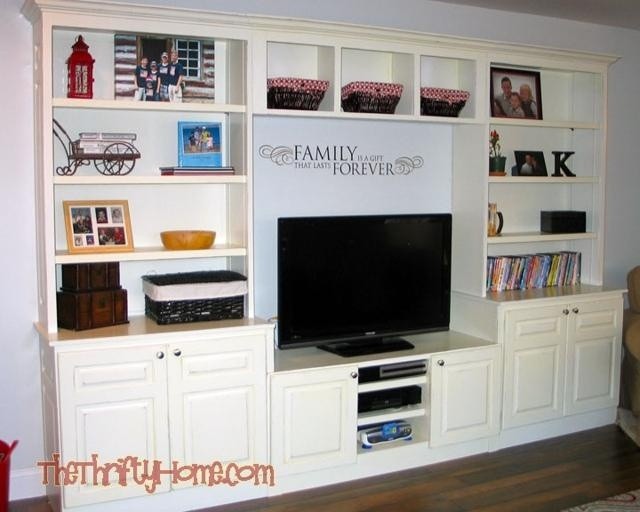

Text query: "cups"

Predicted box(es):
[488, 202, 504, 237]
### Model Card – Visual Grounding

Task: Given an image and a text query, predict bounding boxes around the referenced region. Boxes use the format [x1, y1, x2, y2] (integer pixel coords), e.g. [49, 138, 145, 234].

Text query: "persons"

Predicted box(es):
[72, 208, 122, 247]
[133, 49, 183, 102]
[519, 155, 538, 176]
[188, 124, 216, 153]
[493, 76, 538, 118]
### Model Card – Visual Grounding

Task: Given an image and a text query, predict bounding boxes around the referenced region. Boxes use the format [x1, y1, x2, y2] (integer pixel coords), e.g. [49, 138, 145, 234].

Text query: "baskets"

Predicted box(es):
[267, 78, 330, 110]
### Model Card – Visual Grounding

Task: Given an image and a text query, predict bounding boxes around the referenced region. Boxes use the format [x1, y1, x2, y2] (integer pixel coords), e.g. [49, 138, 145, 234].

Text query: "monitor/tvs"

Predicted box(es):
[275, 212, 455, 358]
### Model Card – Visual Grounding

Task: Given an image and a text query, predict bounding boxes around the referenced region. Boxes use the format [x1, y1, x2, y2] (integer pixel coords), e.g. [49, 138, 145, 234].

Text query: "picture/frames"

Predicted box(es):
[61, 200, 136, 255]
[513, 150, 548, 176]
[177, 121, 222, 168]
[489, 66, 543, 120]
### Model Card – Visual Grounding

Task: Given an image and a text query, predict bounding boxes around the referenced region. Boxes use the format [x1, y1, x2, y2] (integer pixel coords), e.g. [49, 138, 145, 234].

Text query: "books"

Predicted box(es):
[486, 248, 584, 292]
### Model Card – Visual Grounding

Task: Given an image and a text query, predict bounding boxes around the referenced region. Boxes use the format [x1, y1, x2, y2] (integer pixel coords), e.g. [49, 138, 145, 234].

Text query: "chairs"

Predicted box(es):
[622, 263, 639, 419]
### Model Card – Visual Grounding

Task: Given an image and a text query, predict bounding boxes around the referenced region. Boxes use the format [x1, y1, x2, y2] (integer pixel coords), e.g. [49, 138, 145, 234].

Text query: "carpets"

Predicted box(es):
[561, 489, 640, 511]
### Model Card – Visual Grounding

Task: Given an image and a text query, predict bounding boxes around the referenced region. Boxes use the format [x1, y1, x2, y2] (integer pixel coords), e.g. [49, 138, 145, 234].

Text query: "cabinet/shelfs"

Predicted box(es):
[428, 348, 501, 451]
[250, 14, 479, 127]
[353, 356, 432, 458]
[484, 42, 622, 291]
[502, 288, 627, 434]
[34, 332, 269, 510]
[272, 370, 358, 476]
[19, 0, 249, 335]
